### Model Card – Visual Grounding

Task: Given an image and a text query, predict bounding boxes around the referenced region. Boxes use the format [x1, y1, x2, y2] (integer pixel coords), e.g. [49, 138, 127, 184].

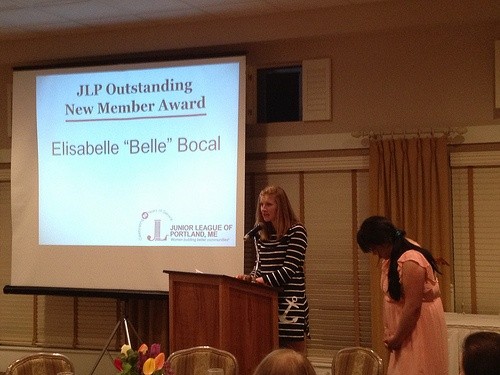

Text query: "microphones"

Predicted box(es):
[243, 221, 264, 240]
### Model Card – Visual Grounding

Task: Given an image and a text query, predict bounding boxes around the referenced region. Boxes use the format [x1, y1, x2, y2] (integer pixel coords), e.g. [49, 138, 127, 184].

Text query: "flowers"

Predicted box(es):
[112, 342, 165, 375]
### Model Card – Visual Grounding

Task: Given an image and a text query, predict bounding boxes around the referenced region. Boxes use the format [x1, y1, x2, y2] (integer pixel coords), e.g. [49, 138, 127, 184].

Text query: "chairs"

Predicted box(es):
[331, 346, 384, 375]
[166, 345, 240, 375]
[4, 353, 75, 375]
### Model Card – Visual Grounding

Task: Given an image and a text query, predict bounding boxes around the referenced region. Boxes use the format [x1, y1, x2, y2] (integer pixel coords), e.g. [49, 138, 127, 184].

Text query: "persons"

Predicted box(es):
[236, 186, 310, 355]
[357, 216, 449, 375]
[462, 332, 500, 375]
